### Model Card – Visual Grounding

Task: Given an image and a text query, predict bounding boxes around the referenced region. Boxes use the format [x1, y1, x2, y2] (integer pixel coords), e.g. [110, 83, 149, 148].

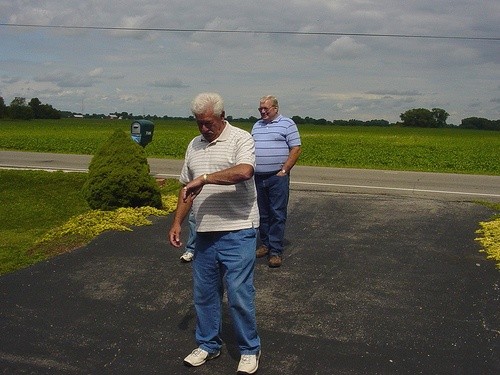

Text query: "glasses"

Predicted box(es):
[258, 105, 275, 112]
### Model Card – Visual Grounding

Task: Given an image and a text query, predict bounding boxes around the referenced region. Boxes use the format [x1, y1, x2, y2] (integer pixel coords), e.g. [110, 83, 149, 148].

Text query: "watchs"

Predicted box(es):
[282, 169, 287, 174]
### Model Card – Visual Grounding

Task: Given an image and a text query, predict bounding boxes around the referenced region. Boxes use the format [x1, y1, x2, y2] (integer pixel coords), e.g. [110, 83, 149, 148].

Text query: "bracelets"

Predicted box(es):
[203, 174, 207, 184]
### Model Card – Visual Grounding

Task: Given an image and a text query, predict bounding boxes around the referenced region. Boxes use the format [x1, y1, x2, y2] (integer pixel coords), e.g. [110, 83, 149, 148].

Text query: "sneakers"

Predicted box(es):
[236, 348, 261, 374]
[184, 347, 221, 366]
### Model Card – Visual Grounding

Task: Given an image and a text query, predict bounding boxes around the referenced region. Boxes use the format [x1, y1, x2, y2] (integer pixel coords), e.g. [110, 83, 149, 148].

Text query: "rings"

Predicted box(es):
[183, 187, 187, 190]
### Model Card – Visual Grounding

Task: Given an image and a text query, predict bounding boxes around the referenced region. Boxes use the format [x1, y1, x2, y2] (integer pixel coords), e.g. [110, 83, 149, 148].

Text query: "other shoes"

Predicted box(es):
[256, 244, 269, 257]
[268, 254, 282, 267]
[180, 252, 194, 261]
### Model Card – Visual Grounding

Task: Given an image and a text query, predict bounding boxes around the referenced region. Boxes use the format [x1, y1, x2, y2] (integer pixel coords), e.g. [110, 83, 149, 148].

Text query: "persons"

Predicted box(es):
[180, 204, 196, 262]
[169, 94, 260, 375]
[251, 94, 301, 267]
